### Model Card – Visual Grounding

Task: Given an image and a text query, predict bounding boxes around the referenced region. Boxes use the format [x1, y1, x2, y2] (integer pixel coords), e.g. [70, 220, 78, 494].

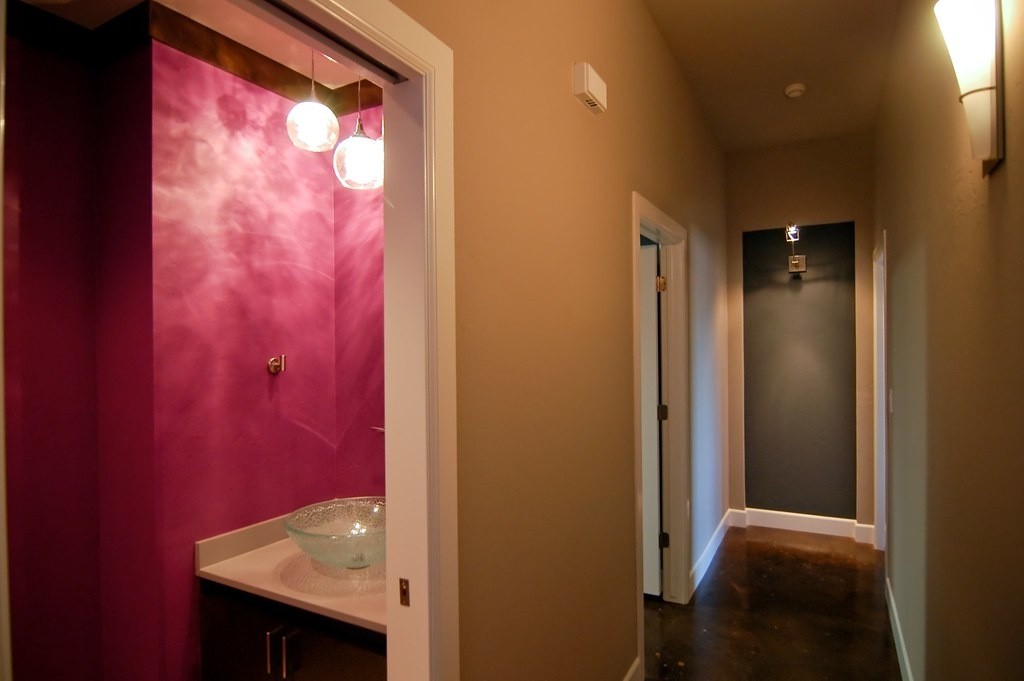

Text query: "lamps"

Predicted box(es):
[928, 0, 1004, 178]
[286, 49, 339, 151]
[785, 222, 800, 264]
[333, 75, 383, 189]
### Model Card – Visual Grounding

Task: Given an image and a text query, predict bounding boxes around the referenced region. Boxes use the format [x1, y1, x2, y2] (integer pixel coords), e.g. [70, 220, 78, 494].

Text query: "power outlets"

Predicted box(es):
[789, 255, 807, 273]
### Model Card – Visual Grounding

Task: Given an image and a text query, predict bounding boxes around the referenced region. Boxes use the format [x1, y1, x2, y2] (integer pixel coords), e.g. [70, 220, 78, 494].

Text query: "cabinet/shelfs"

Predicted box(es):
[199, 577, 386, 681]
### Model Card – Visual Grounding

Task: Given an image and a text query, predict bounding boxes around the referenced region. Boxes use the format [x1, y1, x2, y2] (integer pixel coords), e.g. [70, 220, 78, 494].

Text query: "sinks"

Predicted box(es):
[285, 496, 385, 568]
